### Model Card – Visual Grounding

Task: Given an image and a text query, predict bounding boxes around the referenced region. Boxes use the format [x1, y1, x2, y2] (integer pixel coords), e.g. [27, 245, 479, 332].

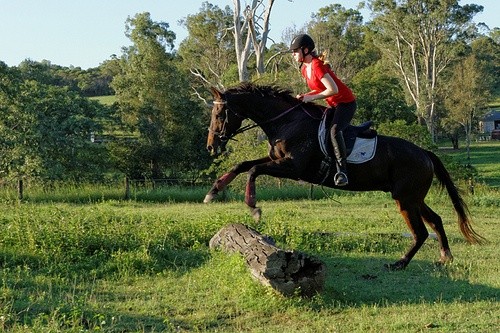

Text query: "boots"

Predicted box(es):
[331, 130, 348, 184]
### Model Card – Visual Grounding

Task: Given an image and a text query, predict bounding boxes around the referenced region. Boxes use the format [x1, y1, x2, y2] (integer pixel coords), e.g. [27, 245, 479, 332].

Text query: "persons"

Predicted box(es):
[290, 33, 358, 184]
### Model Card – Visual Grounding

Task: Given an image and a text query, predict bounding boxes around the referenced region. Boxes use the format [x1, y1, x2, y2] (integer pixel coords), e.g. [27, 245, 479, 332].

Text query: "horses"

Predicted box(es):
[202, 77, 490, 271]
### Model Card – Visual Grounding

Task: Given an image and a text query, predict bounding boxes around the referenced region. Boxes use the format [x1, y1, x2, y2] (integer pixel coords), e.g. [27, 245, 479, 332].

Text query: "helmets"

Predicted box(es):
[290, 34, 315, 56]
